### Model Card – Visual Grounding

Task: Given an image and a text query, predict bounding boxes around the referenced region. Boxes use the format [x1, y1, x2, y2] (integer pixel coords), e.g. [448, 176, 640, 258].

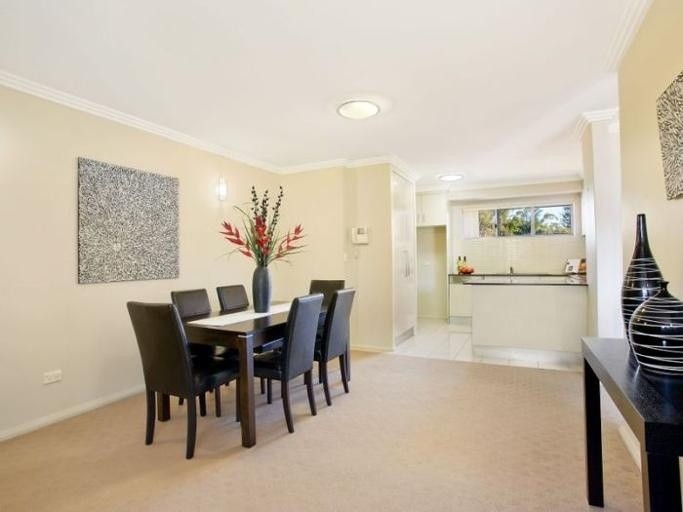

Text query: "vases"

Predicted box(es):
[252, 266, 272, 313]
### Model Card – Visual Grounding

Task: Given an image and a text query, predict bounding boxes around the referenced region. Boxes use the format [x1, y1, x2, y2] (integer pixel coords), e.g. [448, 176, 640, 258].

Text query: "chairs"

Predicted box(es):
[216, 285, 265, 395]
[252, 293, 324, 432]
[281, 288, 357, 405]
[303, 279, 346, 384]
[126, 301, 240, 459]
[171, 289, 229, 416]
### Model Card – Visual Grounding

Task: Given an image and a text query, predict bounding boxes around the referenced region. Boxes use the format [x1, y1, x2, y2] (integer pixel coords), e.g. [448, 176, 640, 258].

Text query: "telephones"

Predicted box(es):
[352, 226, 369, 244]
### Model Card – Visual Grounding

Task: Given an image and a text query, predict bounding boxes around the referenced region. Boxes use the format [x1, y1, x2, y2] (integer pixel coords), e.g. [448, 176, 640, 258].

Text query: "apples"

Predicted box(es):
[460, 265, 473, 273]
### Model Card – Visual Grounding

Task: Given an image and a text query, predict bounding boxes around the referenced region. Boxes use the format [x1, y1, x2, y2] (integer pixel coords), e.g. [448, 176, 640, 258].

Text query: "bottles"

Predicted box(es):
[457, 255, 466, 274]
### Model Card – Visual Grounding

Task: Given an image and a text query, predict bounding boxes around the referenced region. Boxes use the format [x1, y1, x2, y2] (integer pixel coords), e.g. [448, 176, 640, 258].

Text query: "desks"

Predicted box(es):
[179, 300, 329, 448]
[580, 336, 683, 512]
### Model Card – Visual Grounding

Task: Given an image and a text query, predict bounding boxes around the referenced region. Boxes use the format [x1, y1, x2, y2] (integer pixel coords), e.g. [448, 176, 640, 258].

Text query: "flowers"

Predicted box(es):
[218, 184, 307, 267]
